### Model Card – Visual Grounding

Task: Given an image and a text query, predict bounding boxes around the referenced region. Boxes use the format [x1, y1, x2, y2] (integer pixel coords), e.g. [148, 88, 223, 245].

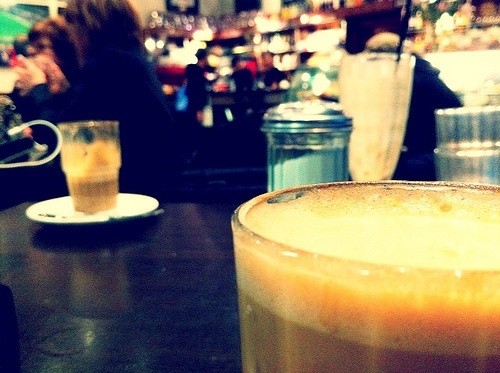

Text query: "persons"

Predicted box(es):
[0, 1, 176, 197]
[336, 13, 464, 181]
[170, 49, 296, 174]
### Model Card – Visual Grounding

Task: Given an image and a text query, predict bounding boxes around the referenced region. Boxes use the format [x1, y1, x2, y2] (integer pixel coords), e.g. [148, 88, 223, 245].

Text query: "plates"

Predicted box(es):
[25, 193, 160, 225]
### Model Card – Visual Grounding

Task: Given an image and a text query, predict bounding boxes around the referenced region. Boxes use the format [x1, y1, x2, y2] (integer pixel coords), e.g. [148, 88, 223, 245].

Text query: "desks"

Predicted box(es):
[0, 198, 242, 373]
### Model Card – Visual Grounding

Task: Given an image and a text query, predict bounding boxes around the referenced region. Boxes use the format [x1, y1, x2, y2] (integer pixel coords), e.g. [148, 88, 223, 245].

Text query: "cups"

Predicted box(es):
[261, 100, 353, 193]
[339, 53, 419, 182]
[57, 119, 122, 213]
[431, 106, 500, 187]
[230, 180, 500, 373]
